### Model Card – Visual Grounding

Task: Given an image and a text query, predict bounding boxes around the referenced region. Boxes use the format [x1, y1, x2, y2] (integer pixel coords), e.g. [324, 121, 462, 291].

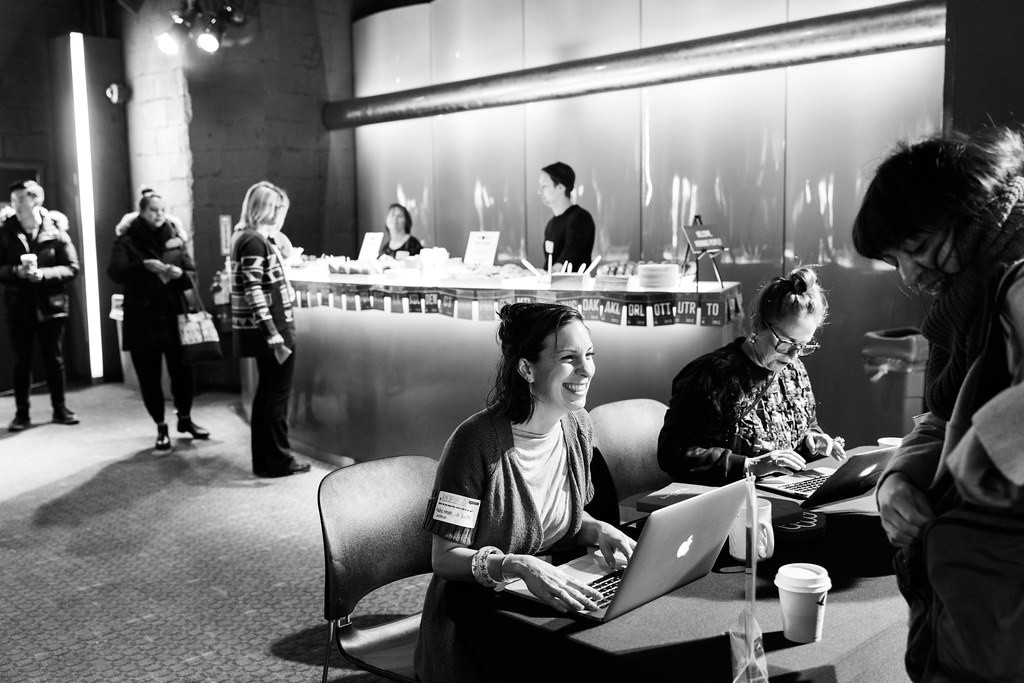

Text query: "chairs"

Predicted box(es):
[318, 456, 437, 682]
[588, 397, 678, 523]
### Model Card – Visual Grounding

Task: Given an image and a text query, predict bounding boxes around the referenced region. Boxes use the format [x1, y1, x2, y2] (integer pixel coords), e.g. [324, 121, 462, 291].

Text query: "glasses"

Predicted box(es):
[758, 313, 822, 358]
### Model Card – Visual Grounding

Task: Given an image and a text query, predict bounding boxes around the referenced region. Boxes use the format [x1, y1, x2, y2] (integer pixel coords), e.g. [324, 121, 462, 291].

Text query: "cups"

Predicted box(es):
[20, 254, 38, 273]
[773, 563, 832, 644]
[591, 274, 632, 292]
[550, 272, 591, 292]
[728, 497, 775, 562]
[877, 437, 903, 448]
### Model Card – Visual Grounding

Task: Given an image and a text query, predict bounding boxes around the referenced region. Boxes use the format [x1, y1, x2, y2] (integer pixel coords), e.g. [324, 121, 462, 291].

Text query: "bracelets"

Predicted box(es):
[471, 545, 504, 588]
[501, 553, 514, 581]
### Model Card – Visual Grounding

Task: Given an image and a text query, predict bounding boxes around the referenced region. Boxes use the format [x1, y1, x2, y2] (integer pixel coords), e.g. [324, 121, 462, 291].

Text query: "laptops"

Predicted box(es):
[509, 477, 757, 621]
[755, 444, 900, 509]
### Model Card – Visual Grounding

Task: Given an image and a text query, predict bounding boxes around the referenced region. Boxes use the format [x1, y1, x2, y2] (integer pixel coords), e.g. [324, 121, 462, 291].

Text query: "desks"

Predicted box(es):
[496, 445, 911, 683]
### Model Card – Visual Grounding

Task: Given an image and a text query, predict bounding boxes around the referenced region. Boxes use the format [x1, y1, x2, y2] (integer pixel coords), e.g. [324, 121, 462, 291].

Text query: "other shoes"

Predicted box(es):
[290, 459, 312, 473]
[155, 423, 171, 450]
[177, 415, 210, 439]
[53, 411, 80, 423]
[8, 416, 29, 430]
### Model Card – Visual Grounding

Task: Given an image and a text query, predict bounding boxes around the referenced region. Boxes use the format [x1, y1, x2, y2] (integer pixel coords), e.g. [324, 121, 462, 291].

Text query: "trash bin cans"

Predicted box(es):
[861, 326, 929, 446]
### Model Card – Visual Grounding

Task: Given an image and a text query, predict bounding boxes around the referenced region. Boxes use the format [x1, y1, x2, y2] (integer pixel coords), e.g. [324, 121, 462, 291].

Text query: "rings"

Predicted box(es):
[774, 458, 779, 465]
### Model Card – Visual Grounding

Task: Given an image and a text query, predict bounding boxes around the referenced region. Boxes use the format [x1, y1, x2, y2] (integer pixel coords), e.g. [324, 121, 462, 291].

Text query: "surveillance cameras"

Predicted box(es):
[106, 83, 129, 104]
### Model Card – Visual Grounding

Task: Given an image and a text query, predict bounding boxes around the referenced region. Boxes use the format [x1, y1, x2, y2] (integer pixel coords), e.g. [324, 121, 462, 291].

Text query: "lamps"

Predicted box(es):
[172, 0, 252, 54]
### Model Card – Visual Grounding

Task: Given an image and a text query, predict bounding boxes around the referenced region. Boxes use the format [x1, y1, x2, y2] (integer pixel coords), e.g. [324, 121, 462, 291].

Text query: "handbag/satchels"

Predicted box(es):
[178, 272, 220, 346]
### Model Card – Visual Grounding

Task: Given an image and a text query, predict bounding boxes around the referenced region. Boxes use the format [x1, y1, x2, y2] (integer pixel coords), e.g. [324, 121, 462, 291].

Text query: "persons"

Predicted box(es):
[230, 180, 312, 478]
[658, 266, 846, 485]
[0, 179, 80, 432]
[106, 188, 210, 452]
[378, 203, 424, 259]
[413, 303, 638, 683]
[851, 130, 1024, 548]
[538, 161, 596, 273]
